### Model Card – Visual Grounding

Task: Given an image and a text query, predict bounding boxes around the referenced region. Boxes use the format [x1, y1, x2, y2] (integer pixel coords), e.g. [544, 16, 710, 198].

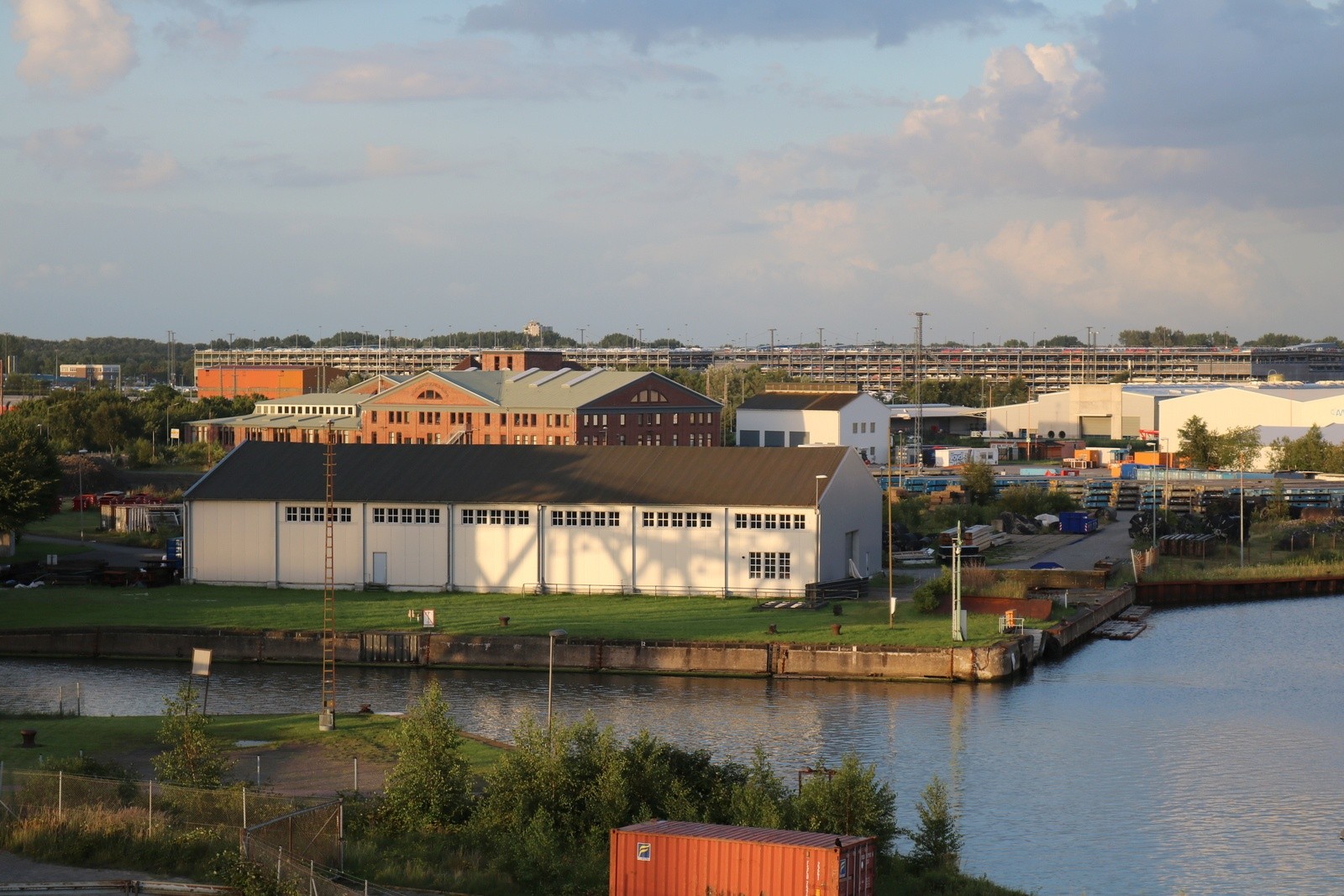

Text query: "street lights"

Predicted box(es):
[1145, 443, 1156, 546]
[1161, 437, 1169, 522]
[547, 629, 568, 758]
[898, 430, 903, 487]
[37, 423, 42, 436]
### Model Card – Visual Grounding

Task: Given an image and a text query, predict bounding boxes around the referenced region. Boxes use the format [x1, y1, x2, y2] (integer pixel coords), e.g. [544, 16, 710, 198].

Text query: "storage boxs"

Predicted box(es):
[609, 818, 876, 896]
[1058, 512, 1098, 534]
